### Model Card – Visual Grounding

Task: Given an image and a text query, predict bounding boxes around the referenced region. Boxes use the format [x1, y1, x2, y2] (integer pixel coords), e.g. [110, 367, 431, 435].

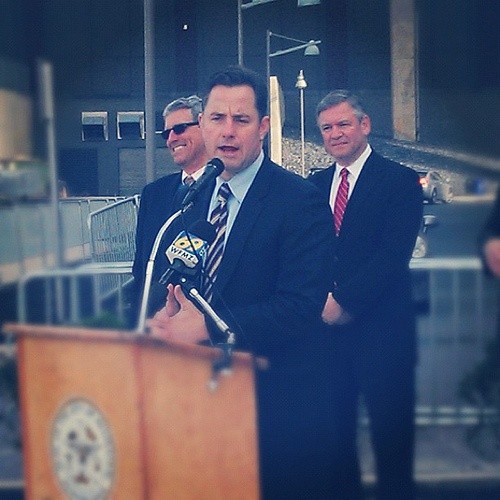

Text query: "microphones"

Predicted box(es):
[179, 157, 224, 204]
[157, 219, 217, 291]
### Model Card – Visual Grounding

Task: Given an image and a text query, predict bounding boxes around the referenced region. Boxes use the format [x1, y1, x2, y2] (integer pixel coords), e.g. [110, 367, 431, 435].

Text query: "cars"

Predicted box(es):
[418, 171, 454, 203]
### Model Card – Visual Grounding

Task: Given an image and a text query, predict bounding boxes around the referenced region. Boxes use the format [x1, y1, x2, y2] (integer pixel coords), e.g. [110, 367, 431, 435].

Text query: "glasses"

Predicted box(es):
[160, 121, 199, 140]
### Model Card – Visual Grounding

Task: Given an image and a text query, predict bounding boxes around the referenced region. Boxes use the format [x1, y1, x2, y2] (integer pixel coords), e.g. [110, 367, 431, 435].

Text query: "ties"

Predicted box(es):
[184, 175, 195, 188]
[198, 183, 232, 304]
[333, 168, 350, 239]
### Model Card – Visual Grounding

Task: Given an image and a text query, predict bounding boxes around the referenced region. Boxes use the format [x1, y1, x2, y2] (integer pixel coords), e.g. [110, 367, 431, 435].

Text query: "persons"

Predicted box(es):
[132, 95, 500, 500]
[152, 67, 350, 500]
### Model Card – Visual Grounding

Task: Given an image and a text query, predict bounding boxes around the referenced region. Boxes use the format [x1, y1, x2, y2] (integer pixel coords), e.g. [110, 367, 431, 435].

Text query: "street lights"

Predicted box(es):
[265, 28, 322, 179]
